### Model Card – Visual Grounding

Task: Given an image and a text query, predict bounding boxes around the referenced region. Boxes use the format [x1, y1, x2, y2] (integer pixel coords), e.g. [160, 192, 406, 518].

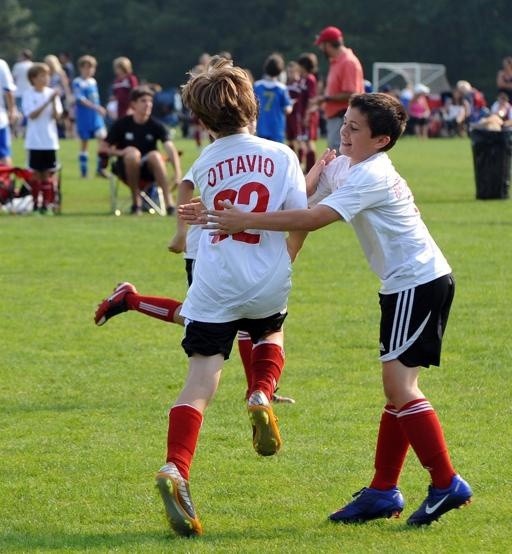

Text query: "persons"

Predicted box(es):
[312, 26, 366, 158]
[155, 59, 310, 542]
[0, 46, 511, 215]
[177, 89, 477, 530]
[93, 96, 339, 404]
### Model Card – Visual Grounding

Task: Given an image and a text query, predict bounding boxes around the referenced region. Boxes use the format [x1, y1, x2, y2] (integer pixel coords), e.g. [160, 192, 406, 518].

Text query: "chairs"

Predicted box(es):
[98, 147, 183, 217]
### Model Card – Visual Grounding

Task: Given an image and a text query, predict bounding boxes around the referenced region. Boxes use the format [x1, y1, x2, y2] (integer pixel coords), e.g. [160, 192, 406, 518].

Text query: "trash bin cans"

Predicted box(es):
[469, 128, 512, 199]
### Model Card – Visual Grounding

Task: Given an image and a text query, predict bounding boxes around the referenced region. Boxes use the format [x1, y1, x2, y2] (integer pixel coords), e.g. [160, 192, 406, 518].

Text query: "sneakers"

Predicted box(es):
[327, 486, 404, 524]
[155, 462, 202, 538]
[245, 390, 295, 456]
[406, 474, 473, 528]
[94, 283, 138, 326]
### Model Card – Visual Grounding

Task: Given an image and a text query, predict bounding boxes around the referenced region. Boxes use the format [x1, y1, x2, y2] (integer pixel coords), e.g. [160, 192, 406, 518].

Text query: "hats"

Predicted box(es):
[314, 26, 342, 45]
